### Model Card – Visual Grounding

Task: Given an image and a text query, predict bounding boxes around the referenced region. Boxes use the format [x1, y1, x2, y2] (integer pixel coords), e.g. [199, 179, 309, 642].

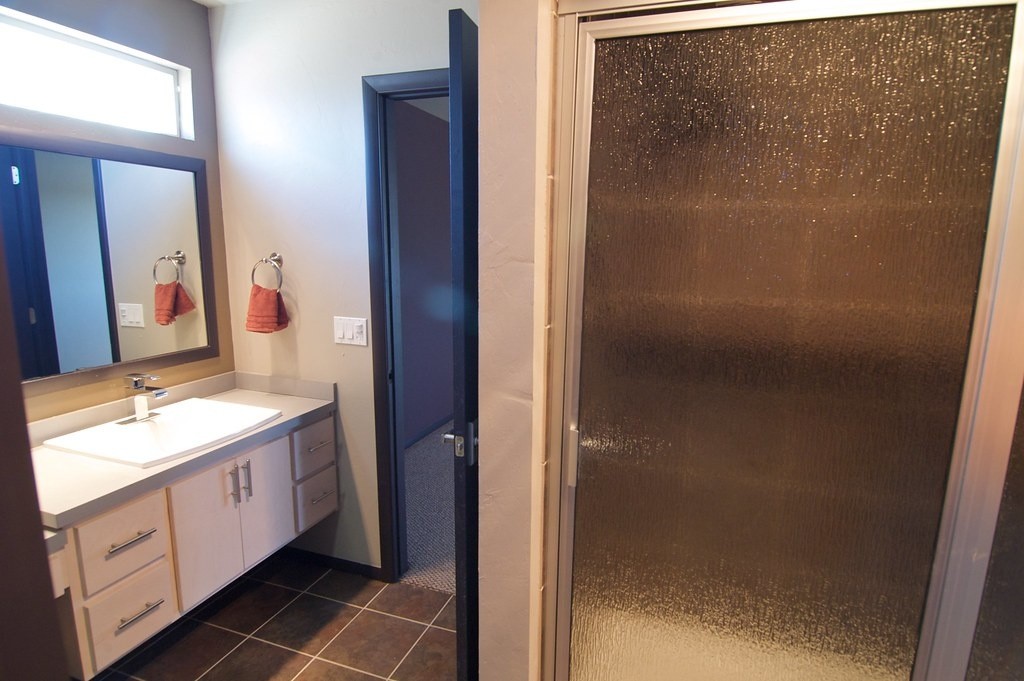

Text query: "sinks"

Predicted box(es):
[41, 395, 285, 470]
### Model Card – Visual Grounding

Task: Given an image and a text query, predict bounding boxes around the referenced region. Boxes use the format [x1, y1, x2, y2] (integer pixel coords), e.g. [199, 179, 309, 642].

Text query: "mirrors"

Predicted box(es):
[6, 143, 117, 382]
[97, 157, 212, 365]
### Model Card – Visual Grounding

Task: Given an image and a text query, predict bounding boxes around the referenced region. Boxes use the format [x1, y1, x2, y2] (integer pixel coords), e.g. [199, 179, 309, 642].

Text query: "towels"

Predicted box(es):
[151, 279, 198, 329]
[243, 284, 291, 337]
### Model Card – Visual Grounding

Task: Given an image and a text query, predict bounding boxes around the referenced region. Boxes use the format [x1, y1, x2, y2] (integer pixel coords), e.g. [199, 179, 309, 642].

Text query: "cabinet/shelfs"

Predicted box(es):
[71, 485, 181, 681]
[165, 434, 298, 617]
[291, 413, 340, 536]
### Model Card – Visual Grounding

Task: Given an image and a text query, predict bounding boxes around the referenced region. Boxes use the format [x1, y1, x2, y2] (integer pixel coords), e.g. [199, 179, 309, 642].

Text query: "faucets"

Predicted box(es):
[122, 372, 171, 424]
